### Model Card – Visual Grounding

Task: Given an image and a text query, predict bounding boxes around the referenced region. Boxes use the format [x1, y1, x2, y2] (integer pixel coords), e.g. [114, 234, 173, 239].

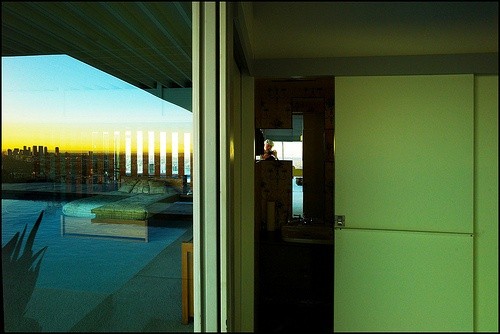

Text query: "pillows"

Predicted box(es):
[148, 181, 167, 194]
[117, 180, 137, 193]
[130, 179, 149, 194]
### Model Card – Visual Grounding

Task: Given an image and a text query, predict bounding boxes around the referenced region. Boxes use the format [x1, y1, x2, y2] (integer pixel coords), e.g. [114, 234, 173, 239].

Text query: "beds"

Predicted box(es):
[60, 191, 180, 243]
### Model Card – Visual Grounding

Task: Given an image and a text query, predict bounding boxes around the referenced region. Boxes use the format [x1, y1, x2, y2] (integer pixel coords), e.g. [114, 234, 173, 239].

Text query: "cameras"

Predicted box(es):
[265, 149, 274, 155]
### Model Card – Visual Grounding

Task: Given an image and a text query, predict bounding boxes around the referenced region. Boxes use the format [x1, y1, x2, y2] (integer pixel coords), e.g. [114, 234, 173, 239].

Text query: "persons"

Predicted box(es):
[260, 139, 279, 161]
